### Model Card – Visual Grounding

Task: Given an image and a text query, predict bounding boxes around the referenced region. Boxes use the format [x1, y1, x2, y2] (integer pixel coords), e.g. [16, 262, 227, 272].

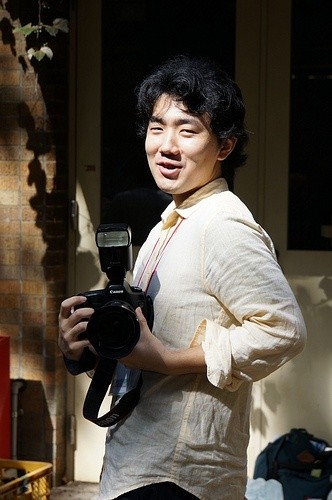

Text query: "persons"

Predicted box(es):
[58, 52, 307, 500]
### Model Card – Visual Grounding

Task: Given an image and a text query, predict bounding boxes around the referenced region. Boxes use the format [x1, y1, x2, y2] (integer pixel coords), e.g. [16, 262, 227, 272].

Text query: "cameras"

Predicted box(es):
[74, 223, 154, 359]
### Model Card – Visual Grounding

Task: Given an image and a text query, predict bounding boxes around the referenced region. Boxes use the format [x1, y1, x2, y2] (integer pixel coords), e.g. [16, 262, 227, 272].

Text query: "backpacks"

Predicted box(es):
[253, 427, 332, 500]
